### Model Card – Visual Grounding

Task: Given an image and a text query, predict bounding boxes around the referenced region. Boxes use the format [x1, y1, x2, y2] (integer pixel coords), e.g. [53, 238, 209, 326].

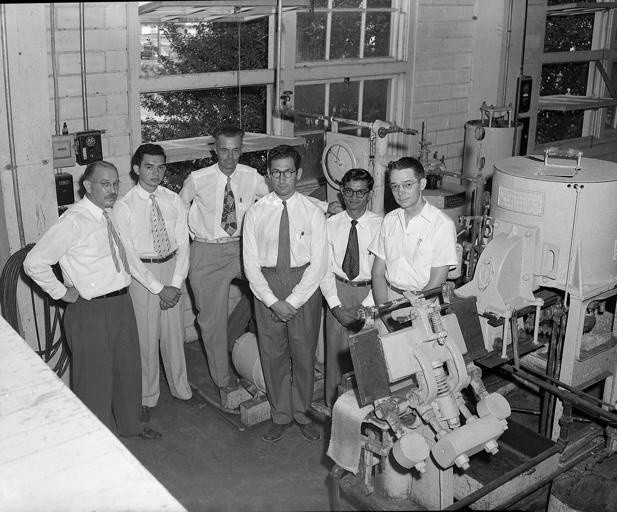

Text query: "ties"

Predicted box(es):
[275, 200, 293, 287]
[149, 194, 172, 260]
[342, 220, 360, 281]
[220, 176, 238, 236]
[102, 209, 133, 276]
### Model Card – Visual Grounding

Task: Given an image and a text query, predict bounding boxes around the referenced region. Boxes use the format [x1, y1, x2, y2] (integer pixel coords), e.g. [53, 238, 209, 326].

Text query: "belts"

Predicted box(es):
[140, 250, 177, 263]
[261, 262, 309, 273]
[335, 273, 372, 287]
[385, 277, 407, 295]
[194, 236, 244, 245]
[94, 286, 128, 299]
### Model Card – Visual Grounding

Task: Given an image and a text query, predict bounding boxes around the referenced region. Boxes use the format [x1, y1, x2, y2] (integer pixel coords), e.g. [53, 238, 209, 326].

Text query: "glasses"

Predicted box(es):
[342, 187, 370, 199]
[389, 180, 420, 191]
[89, 179, 122, 189]
[269, 167, 297, 179]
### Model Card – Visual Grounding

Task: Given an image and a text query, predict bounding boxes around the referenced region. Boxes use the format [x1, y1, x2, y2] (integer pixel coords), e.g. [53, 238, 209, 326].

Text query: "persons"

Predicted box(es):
[320, 168, 384, 415]
[115, 144, 207, 423]
[243, 145, 327, 443]
[177, 126, 270, 391]
[367, 156, 459, 332]
[23, 160, 162, 447]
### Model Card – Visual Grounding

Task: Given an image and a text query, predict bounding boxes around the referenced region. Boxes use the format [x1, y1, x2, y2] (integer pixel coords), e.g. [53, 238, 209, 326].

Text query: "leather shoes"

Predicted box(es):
[259, 417, 295, 445]
[141, 404, 154, 420]
[139, 427, 161, 442]
[294, 414, 324, 444]
[169, 390, 209, 411]
